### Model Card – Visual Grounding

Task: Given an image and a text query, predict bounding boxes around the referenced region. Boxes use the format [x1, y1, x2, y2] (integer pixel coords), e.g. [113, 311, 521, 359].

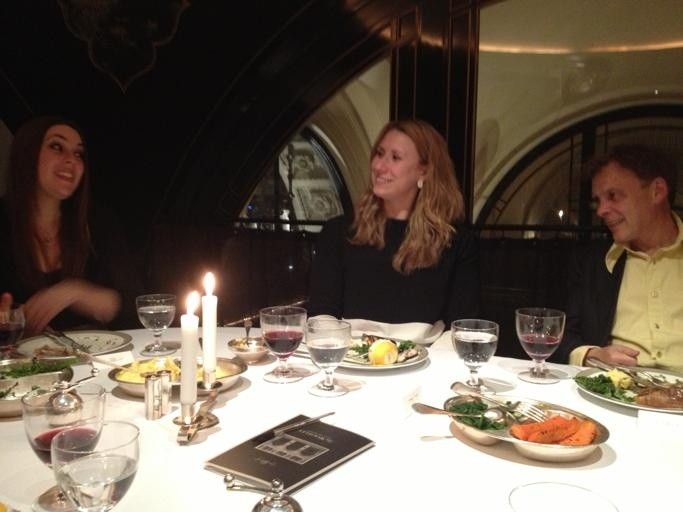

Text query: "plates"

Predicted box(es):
[444, 395, 611, 464]
[1, 330, 248, 417]
[342, 336, 429, 370]
[573, 366, 683, 413]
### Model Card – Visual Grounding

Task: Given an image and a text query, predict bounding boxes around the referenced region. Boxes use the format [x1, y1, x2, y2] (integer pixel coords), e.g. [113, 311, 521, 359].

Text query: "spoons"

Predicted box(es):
[412, 402, 507, 425]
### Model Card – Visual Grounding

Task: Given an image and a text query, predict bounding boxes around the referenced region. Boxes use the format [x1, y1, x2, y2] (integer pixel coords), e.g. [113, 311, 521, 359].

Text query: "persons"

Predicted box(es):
[307, 119, 483, 331]
[0, 114, 147, 337]
[559, 144, 683, 374]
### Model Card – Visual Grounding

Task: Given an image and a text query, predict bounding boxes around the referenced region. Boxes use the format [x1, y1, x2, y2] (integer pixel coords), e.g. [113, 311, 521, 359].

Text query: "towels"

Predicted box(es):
[307, 313, 447, 344]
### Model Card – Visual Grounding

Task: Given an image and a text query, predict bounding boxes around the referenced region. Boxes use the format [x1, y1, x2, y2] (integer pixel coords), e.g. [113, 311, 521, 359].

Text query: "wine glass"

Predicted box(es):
[20, 382, 140, 512]
[260, 306, 349, 398]
[135, 294, 177, 356]
[450, 308, 567, 394]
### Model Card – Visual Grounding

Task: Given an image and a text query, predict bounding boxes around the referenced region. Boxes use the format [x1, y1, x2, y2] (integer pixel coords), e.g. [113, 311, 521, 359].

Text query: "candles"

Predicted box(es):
[180, 291, 199, 404]
[202, 272, 218, 372]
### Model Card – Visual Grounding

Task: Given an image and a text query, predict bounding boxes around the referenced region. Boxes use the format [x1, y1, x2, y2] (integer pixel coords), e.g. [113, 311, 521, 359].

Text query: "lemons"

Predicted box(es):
[368, 338, 398, 365]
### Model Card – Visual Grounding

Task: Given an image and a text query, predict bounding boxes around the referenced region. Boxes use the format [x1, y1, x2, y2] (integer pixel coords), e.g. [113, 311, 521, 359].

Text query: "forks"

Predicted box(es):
[450, 381, 548, 423]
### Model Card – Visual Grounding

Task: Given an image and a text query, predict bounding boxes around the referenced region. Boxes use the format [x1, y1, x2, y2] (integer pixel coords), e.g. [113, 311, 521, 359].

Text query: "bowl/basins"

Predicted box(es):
[227, 336, 267, 364]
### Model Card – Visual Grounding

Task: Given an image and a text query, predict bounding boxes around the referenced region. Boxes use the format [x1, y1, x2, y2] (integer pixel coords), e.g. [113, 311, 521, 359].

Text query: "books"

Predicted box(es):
[203, 414, 376, 493]
